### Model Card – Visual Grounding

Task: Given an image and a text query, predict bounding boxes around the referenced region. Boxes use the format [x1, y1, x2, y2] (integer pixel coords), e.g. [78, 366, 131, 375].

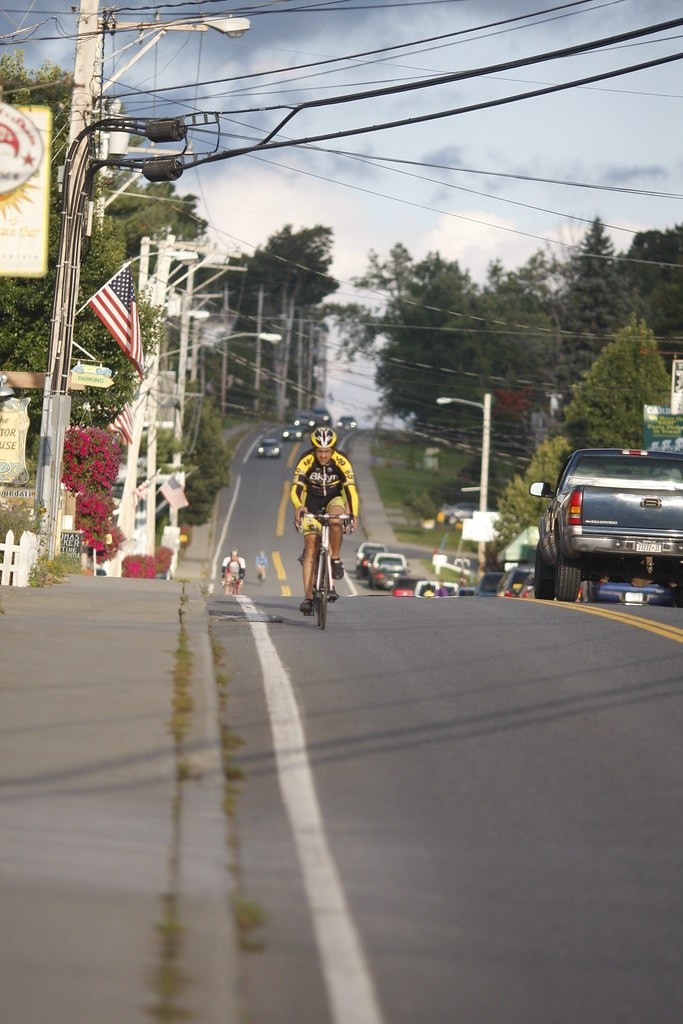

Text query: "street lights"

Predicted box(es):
[110, 333, 284, 577]
[435, 391, 493, 582]
[34, 12, 253, 560]
[144, 307, 209, 557]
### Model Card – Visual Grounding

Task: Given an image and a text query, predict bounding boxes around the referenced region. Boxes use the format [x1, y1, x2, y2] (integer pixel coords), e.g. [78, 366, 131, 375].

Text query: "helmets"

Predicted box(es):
[230, 550, 238, 559]
[310, 427, 337, 448]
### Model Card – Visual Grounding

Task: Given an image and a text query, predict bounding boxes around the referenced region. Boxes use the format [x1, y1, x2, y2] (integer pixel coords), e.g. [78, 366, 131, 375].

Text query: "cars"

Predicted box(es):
[577, 578, 674, 609]
[353, 541, 535, 599]
[437, 501, 479, 525]
[257, 438, 282, 458]
[335, 416, 357, 431]
[282, 409, 333, 442]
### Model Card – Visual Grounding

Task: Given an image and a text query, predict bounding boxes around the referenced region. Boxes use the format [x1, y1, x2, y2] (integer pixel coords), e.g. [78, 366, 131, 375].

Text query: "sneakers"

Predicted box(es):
[330, 558, 344, 579]
[299, 599, 312, 613]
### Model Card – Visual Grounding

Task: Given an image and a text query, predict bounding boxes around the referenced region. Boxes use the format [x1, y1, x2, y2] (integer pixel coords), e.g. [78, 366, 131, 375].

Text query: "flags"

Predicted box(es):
[107, 402, 135, 446]
[134, 480, 150, 507]
[162, 477, 188, 509]
[91, 265, 145, 378]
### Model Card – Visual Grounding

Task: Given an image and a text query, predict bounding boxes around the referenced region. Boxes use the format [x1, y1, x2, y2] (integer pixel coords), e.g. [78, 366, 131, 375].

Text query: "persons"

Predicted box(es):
[423, 583, 435, 597]
[255, 551, 267, 578]
[221, 551, 246, 592]
[289, 426, 358, 613]
[434, 580, 448, 596]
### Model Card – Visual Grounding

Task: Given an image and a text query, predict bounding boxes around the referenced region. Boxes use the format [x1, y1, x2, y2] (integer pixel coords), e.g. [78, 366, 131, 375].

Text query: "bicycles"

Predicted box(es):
[293, 507, 355, 629]
[220, 575, 242, 596]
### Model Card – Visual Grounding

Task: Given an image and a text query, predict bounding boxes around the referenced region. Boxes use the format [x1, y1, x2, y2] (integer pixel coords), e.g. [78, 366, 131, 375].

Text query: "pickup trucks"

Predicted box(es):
[527, 446, 683, 608]
